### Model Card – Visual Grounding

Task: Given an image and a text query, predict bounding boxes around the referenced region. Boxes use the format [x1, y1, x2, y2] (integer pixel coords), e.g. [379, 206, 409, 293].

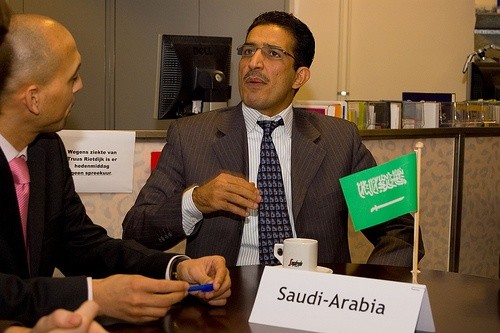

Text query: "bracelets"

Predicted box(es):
[171, 259, 186, 280]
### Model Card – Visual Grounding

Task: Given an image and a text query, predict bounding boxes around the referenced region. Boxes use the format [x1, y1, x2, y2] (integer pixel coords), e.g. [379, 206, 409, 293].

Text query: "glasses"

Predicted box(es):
[237, 46, 296, 60]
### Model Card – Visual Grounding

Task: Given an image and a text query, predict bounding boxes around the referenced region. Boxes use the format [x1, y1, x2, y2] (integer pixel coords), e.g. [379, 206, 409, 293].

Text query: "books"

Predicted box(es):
[295, 92, 456, 129]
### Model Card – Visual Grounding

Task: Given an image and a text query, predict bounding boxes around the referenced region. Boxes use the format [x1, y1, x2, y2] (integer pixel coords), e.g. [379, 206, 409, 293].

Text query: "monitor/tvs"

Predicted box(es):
[155, 32, 233, 117]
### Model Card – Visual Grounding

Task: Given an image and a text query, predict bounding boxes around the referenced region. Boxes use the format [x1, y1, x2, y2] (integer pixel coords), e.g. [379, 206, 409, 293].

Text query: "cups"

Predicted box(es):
[273, 237, 318, 272]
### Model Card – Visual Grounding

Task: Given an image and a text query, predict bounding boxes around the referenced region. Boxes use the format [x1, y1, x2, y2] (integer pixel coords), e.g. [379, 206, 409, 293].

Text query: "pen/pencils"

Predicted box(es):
[188, 283, 215, 291]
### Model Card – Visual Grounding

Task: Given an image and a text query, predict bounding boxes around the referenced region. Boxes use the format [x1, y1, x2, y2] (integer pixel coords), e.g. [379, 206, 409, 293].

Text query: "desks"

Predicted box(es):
[102, 263, 500, 333]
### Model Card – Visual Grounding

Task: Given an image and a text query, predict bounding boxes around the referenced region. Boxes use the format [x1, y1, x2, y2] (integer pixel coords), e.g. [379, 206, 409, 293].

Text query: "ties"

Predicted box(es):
[257, 118, 293, 265]
[8, 158, 30, 251]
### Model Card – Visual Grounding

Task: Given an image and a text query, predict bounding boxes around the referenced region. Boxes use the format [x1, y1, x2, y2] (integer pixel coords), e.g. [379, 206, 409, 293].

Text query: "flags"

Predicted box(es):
[339, 152, 418, 232]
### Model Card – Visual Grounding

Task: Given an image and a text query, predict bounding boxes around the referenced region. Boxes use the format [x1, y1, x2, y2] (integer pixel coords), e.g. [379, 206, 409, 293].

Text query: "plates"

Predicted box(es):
[277, 265, 333, 274]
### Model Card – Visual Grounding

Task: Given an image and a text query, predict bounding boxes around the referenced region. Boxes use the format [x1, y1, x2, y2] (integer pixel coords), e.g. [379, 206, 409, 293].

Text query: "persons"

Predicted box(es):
[123, 11, 425, 267]
[0, 14, 232, 322]
[0, 0, 108, 333]
